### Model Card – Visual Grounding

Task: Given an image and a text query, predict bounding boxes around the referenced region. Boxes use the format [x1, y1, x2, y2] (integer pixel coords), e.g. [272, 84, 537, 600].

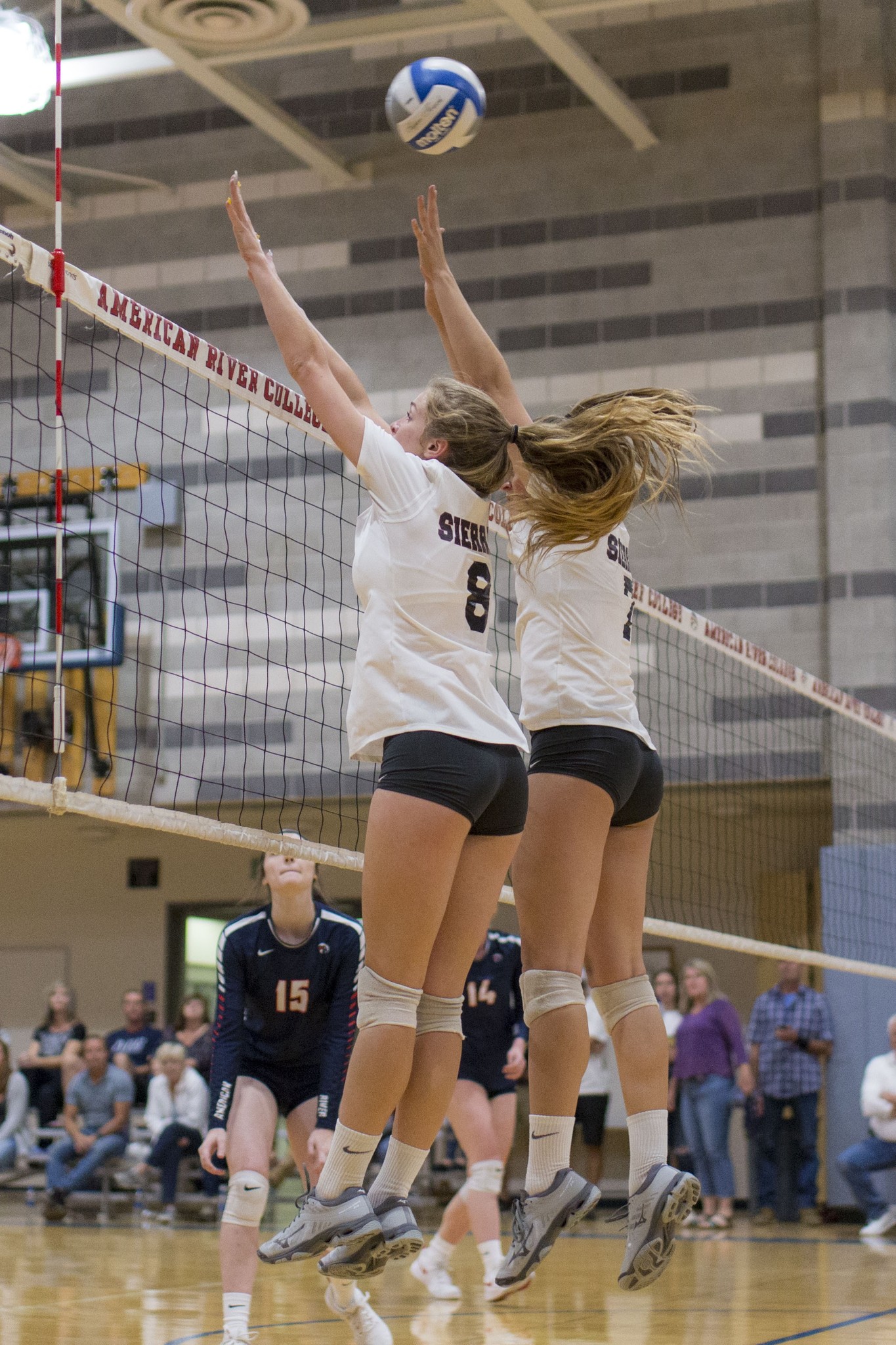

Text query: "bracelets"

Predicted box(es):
[796, 1031, 813, 1053]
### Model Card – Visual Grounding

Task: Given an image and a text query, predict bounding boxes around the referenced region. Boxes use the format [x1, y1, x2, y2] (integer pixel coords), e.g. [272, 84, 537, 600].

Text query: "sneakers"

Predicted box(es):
[220, 1324, 259, 1345]
[318, 1195, 425, 1281]
[495, 1167, 601, 1288]
[604, 1162, 700, 1291]
[324, 1284, 395, 1345]
[256, 1163, 382, 1264]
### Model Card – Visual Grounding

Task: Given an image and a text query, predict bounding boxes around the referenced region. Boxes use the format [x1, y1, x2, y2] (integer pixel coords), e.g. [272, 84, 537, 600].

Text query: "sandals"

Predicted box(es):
[696, 1212, 734, 1228]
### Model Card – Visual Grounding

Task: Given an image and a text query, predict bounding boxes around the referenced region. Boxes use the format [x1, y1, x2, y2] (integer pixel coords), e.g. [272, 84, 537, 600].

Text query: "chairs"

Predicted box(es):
[0, 1109, 469, 1222]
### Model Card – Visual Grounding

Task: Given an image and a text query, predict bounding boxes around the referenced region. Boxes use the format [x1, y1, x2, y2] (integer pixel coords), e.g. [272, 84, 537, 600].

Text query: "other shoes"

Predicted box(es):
[800, 1207, 821, 1225]
[484, 1259, 536, 1303]
[410, 1248, 461, 1300]
[411, 1301, 461, 1342]
[37, 1187, 70, 1222]
[143, 1206, 172, 1221]
[859, 1206, 896, 1237]
[483, 1310, 535, 1344]
[114, 1166, 153, 1191]
[751, 1209, 775, 1227]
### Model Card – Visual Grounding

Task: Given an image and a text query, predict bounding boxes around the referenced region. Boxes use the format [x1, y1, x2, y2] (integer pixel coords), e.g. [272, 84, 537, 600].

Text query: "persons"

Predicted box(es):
[410, 182, 702, 1305]
[198, 829, 395, 1345]
[749, 946, 835, 1228]
[409, 930, 535, 1305]
[225, 171, 732, 1275]
[369, 1103, 514, 1213]
[676, 959, 756, 1230]
[0, 980, 297, 1226]
[654, 969, 685, 1166]
[577, 971, 611, 1220]
[837, 1017, 896, 1237]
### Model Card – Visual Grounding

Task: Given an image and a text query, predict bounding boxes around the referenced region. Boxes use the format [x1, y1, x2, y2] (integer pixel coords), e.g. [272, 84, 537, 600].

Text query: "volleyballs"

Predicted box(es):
[384, 56, 487, 156]
